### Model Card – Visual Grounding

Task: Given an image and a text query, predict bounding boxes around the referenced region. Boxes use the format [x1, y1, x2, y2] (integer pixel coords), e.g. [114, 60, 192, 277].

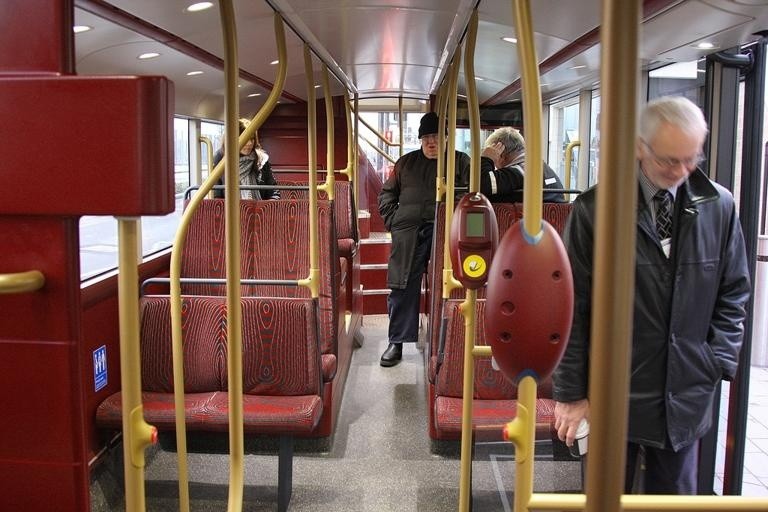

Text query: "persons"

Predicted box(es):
[375, 111, 471, 369]
[553, 92, 754, 498]
[211, 116, 284, 203]
[478, 124, 568, 202]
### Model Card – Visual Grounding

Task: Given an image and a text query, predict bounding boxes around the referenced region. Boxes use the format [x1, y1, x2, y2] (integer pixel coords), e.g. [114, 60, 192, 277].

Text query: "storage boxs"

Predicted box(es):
[357, 209, 371, 240]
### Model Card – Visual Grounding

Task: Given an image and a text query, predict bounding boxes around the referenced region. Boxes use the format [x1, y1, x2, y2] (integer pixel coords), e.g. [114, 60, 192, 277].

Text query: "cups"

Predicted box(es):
[563, 417, 589, 457]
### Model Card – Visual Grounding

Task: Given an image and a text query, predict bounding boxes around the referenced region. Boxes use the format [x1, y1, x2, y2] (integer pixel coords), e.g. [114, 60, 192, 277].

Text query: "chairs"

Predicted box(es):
[180, 180, 356, 375]
[222, 293, 323, 510]
[98, 294, 223, 509]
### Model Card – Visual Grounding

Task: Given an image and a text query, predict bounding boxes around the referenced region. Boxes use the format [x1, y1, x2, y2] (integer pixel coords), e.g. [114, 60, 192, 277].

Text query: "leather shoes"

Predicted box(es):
[380, 341, 403, 367]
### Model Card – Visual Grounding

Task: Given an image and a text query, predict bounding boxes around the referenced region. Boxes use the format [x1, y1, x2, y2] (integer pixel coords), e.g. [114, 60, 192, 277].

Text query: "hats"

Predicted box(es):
[418, 112, 448, 139]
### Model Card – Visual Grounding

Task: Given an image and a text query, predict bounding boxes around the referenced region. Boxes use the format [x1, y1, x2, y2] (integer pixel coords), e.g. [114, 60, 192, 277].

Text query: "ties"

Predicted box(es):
[654, 188, 673, 241]
